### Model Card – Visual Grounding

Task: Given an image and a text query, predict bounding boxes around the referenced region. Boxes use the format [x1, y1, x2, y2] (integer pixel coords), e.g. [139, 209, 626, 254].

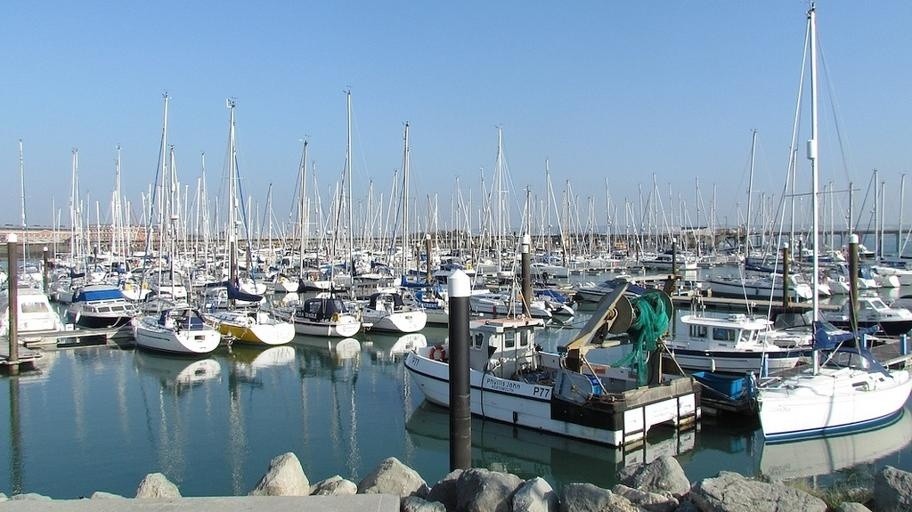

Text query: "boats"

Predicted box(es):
[402, 274, 703, 450]
[402, 401, 702, 487]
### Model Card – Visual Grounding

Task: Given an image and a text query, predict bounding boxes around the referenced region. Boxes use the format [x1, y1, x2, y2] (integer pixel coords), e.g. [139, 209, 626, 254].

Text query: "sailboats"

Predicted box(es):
[349, 119, 428, 336]
[748, 1, 912, 446]
[128, 90, 221, 353]
[749, 405, 912, 490]
[204, 98, 297, 348]
[266, 89, 361, 339]
[402, 401, 702, 487]
[0, 84, 912, 329]
